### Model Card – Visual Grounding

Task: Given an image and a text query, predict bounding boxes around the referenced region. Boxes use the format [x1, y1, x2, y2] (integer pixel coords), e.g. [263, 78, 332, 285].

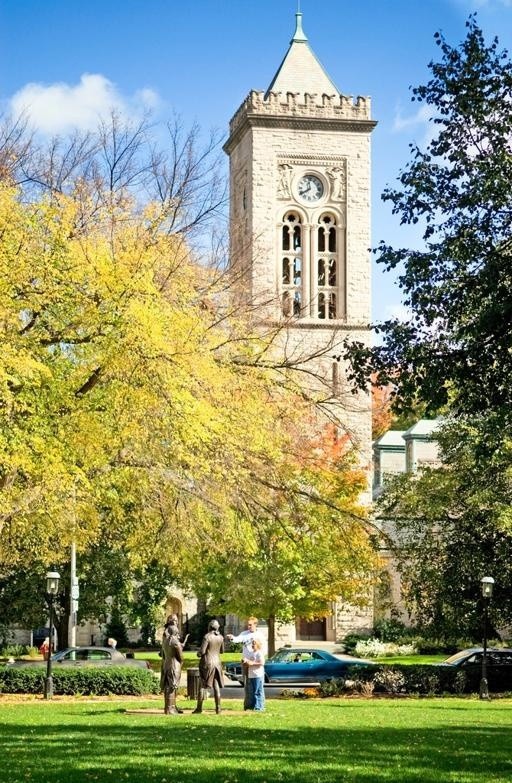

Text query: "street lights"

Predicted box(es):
[480, 577, 495, 699]
[44, 570, 60, 699]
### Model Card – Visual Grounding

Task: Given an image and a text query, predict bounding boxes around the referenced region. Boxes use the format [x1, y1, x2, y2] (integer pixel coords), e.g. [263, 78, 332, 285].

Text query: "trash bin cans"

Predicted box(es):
[187, 668, 207, 700]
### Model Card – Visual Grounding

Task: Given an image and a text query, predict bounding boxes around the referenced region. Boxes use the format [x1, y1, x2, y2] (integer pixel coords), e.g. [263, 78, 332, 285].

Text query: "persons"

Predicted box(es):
[238, 638, 269, 713]
[160, 626, 187, 716]
[162, 614, 192, 650]
[227, 614, 259, 709]
[294, 652, 302, 661]
[41, 636, 51, 660]
[191, 620, 226, 714]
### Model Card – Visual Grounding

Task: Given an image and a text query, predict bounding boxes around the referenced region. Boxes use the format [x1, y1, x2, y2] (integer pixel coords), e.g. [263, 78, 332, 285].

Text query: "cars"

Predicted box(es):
[8, 646, 152, 670]
[224, 647, 376, 687]
[410, 648, 512, 693]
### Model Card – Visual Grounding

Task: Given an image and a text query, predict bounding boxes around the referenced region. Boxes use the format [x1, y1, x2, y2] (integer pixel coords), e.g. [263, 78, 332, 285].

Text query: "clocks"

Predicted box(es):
[291, 167, 330, 209]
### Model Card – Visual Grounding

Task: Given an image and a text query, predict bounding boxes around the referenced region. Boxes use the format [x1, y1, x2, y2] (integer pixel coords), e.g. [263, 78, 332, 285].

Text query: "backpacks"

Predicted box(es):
[40, 644, 47, 654]
